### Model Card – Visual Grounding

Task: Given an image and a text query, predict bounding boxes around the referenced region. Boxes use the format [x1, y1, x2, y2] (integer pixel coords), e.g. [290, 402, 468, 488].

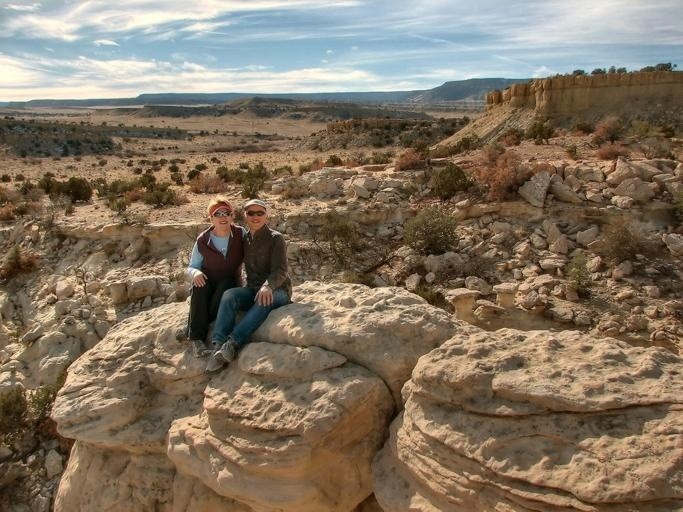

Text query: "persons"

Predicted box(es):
[187, 195, 246, 356]
[205, 199, 291, 371]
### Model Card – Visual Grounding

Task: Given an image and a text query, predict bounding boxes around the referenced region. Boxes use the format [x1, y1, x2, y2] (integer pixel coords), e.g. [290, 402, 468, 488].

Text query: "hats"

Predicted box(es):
[243, 199, 266, 209]
[211, 203, 232, 215]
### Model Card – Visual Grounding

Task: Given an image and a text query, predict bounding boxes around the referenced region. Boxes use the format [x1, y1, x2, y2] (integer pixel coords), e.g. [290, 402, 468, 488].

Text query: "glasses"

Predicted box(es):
[246, 210, 265, 216]
[214, 211, 231, 216]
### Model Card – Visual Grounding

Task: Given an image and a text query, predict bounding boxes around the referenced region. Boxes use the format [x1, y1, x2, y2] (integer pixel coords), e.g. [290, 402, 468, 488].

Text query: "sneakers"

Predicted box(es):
[176, 326, 236, 373]
